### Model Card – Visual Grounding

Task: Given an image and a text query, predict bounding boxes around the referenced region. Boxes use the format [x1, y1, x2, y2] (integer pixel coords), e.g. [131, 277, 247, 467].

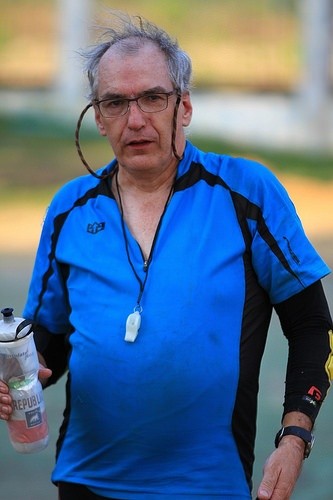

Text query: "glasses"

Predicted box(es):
[94, 89, 178, 118]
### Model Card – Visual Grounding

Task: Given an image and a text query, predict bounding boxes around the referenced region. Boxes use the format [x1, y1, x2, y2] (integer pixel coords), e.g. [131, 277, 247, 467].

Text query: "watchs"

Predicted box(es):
[276, 425, 317, 460]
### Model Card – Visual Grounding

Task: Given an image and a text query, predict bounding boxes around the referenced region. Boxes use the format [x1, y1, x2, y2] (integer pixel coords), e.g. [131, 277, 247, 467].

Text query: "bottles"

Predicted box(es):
[0, 307, 50, 453]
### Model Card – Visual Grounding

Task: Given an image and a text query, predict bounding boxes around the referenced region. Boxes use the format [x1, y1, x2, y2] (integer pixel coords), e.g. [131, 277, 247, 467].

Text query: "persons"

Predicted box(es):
[0, 3, 333, 500]
